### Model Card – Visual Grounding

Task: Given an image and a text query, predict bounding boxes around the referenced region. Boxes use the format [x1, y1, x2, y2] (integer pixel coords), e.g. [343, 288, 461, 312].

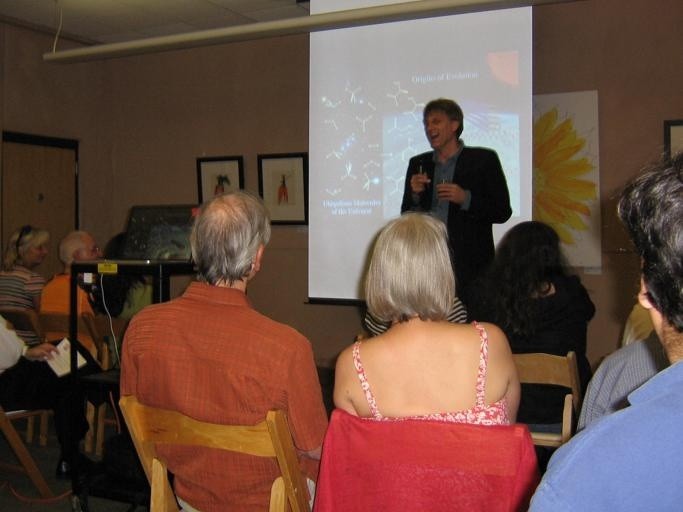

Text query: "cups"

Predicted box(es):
[437, 175, 451, 198]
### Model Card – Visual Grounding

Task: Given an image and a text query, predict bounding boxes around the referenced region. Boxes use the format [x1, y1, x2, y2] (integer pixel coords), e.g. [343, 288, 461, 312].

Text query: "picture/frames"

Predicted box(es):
[195, 151, 308, 226]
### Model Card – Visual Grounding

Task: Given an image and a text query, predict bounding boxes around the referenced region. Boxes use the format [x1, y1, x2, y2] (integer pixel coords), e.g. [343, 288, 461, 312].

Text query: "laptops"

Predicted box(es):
[75, 204, 199, 266]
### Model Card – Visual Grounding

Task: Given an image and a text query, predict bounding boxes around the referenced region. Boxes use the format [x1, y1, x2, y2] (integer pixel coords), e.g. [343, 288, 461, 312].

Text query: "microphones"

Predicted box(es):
[417, 160, 425, 173]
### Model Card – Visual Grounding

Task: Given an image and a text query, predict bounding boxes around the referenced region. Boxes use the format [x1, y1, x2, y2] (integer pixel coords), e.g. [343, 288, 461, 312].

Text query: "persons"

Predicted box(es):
[620, 299, 655, 351]
[473, 220, 597, 477]
[526, 150, 682, 512]
[332, 211, 522, 428]
[0, 222, 50, 346]
[118, 186, 330, 512]
[399, 96, 513, 323]
[39, 229, 107, 361]
[569, 326, 671, 435]
[0, 313, 115, 480]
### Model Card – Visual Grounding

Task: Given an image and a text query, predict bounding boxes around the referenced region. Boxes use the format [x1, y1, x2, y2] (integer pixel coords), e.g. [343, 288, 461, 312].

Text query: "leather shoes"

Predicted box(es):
[55, 453, 107, 481]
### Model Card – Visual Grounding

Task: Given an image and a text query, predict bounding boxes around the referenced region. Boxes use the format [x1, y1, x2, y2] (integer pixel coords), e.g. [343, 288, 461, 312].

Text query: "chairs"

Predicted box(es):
[0, 303, 128, 499]
[511, 348, 584, 448]
[116, 395, 311, 512]
